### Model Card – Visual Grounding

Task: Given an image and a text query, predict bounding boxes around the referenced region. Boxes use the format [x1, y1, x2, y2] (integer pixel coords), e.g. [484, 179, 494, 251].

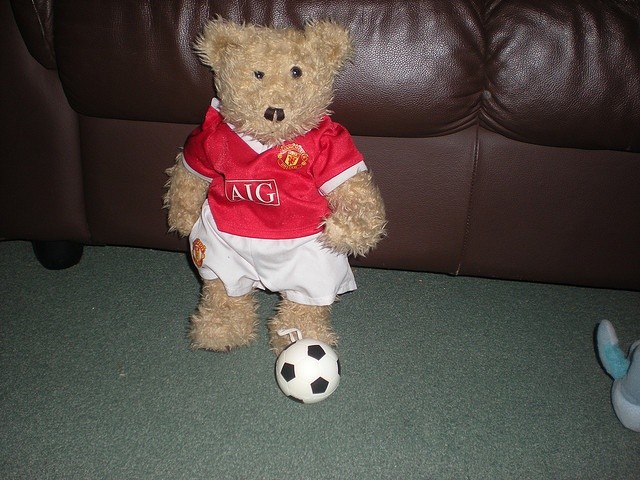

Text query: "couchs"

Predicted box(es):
[1, 1, 638, 292]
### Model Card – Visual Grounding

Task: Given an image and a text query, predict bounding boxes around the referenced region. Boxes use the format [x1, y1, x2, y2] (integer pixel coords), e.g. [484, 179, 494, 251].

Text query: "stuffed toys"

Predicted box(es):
[597, 318, 640, 434]
[161, 12, 387, 351]
